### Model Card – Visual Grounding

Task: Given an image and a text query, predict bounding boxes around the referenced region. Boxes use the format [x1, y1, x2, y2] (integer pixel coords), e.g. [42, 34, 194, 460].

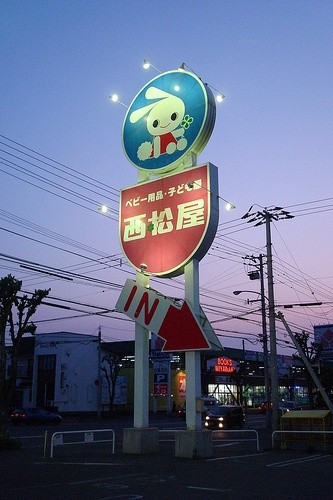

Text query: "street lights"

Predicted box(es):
[231, 289, 281, 433]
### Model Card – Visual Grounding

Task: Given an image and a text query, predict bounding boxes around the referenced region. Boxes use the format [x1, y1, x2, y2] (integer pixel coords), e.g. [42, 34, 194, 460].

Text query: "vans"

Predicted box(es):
[204, 404, 246, 430]
[177, 396, 222, 417]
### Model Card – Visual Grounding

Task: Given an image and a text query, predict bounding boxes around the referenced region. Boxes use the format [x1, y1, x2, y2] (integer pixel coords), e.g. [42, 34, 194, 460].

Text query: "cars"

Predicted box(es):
[9, 407, 64, 426]
[259, 401, 302, 416]
